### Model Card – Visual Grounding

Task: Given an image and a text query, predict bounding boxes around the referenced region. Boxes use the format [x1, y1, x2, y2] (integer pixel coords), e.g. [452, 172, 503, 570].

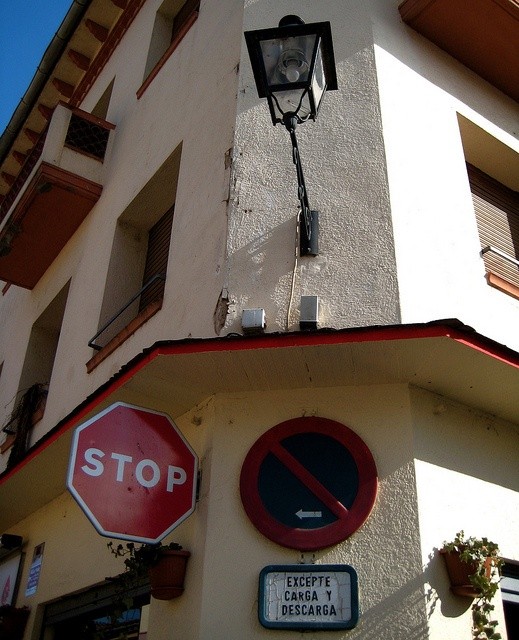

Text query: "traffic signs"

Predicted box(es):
[257, 564, 358, 631]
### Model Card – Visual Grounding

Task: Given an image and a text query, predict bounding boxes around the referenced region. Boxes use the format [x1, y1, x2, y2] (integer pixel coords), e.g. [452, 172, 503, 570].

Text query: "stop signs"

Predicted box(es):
[65, 402, 199, 545]
[238, 416, 378, 552]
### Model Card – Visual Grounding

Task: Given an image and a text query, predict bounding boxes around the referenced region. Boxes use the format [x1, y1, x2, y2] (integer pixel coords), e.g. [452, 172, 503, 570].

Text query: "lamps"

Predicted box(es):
[245, 14, 339, 257]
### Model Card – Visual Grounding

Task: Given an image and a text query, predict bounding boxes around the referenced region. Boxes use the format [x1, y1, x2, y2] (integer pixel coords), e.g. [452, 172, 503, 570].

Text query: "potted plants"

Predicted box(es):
[434, 533, 510, 635]
